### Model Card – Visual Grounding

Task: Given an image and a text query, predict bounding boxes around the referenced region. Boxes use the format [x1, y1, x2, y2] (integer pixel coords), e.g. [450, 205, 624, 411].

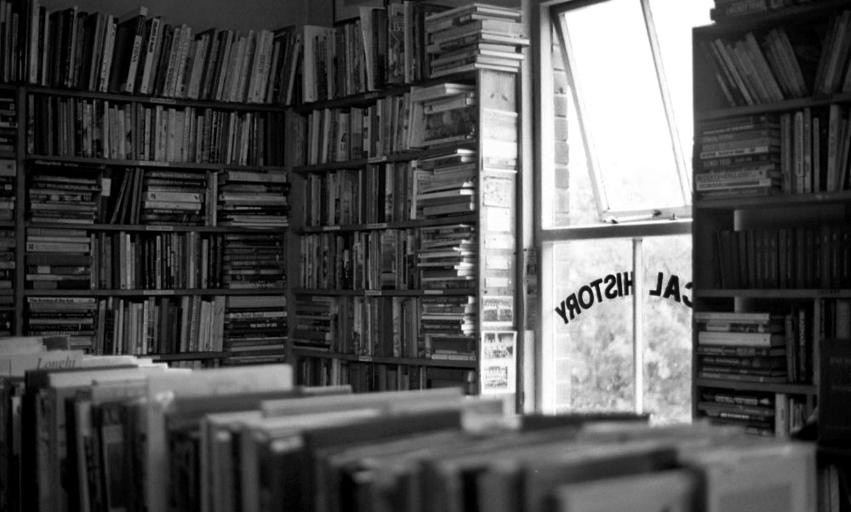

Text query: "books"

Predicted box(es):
[692, 28, 851, 436]
[0, 0, 480, 394]
[1, 336, 817, 512]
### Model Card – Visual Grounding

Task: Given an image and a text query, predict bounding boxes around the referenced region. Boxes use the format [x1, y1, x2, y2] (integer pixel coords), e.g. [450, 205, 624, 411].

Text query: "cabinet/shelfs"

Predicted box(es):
[687, 2, 851, 457]
